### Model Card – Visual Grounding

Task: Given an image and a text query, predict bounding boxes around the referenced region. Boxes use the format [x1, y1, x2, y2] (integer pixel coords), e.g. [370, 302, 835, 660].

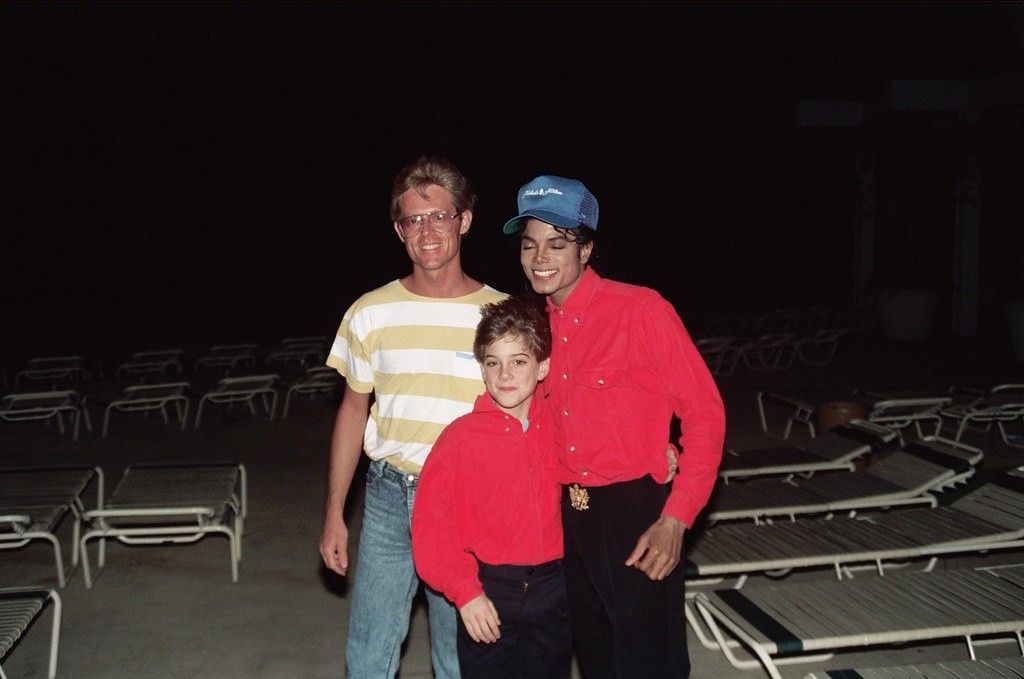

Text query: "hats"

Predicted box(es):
[501, 177, 599, 234]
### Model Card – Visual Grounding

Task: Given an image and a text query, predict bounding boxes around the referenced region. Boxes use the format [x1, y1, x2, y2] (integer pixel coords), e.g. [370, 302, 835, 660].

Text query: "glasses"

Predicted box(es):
[396, 210, 462, 238]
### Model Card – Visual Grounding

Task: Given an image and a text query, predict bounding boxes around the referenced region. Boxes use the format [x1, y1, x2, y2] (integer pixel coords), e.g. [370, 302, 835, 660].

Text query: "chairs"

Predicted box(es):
[0, 341, 1024, 679]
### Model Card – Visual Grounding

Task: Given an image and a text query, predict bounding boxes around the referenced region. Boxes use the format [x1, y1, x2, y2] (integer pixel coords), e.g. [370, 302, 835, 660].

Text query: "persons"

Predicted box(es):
[317, 156, 728, 679]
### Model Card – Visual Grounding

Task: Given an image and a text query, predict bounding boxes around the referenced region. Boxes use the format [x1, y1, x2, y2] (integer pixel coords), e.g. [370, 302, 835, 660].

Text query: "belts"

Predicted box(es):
[559, 474, 656, 511]
